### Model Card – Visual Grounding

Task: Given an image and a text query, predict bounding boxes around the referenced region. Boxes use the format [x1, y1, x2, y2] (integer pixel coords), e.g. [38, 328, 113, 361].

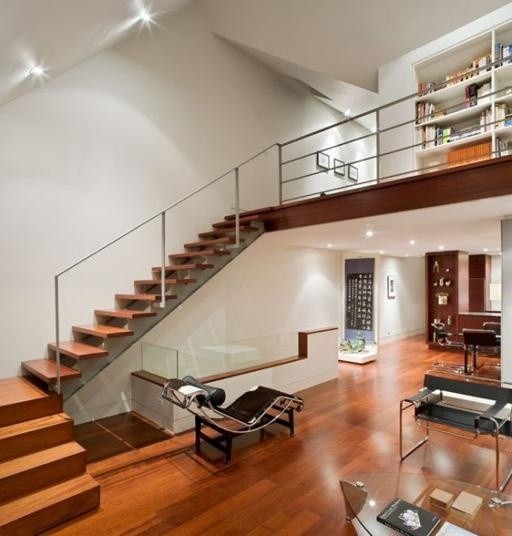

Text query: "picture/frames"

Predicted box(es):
[334, 158, 345, 176]
[348, 164, 358, 181]
[317, 151, 330, 169]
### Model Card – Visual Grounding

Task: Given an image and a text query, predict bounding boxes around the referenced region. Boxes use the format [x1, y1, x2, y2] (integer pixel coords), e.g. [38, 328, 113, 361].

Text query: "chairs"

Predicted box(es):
[162, 376, 305, 465]
[463, 330, 501, 373]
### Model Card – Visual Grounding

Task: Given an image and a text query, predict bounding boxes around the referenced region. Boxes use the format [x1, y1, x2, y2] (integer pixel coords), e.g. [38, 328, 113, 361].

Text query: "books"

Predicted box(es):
[376, 496, 442, 536]
[415, 39, 511, 159]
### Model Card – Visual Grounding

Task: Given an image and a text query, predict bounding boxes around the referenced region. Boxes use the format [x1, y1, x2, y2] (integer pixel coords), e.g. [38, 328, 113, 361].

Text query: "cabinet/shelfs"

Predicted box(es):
[410, 19, 511, 175]
[425, 251, 501, 344]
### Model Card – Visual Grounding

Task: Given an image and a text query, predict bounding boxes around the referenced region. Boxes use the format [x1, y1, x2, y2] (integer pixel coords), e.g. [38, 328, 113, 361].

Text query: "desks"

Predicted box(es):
[399, 386, 512, 492]
